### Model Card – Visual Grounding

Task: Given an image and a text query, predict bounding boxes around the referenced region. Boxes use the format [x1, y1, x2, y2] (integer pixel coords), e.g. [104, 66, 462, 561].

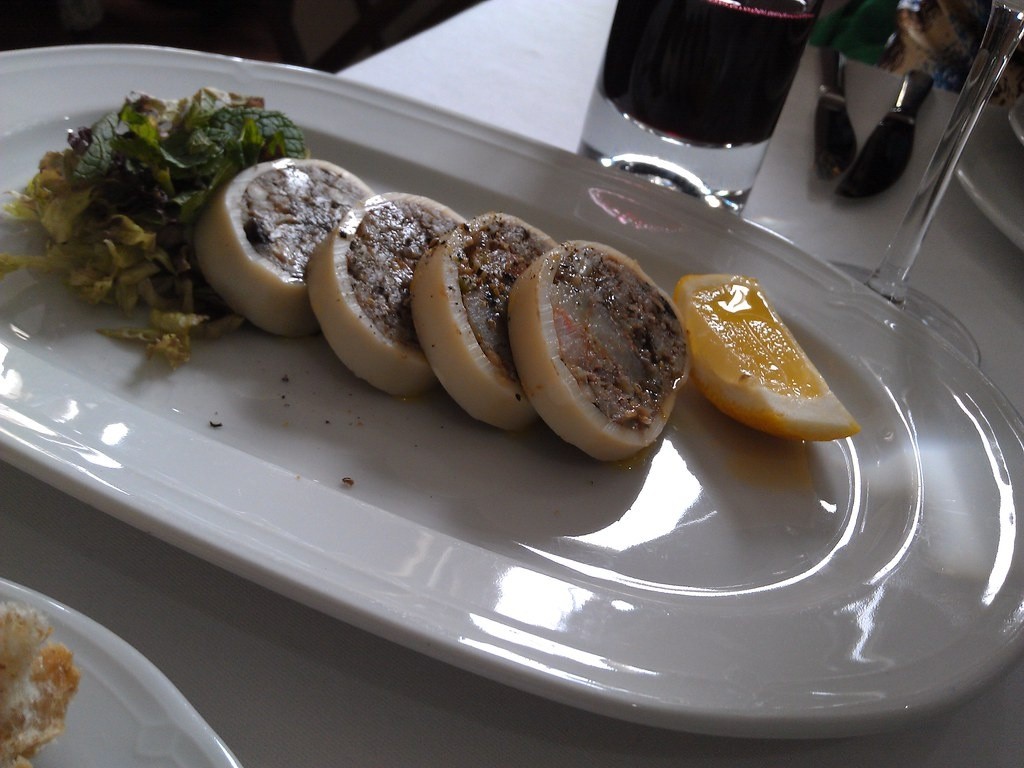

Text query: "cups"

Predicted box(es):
[578, 0, 825, 216]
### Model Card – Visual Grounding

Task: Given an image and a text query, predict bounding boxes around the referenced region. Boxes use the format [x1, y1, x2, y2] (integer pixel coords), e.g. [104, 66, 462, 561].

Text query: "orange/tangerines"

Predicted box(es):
[674, 273, 794, 427]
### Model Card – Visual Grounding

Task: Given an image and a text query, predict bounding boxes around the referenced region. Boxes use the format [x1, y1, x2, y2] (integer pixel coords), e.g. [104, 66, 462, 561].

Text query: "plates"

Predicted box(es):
[1, 43, 1024, 737]
[1, 578, 244, 767]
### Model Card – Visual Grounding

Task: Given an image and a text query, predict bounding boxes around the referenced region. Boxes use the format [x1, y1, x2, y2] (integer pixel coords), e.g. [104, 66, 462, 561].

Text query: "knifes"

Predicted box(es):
[834, 68, 934, 198]
[815, 47, 856, 181]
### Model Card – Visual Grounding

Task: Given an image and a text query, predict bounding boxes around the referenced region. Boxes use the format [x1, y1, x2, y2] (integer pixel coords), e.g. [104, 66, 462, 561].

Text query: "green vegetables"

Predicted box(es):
[0, 85, 313, 369]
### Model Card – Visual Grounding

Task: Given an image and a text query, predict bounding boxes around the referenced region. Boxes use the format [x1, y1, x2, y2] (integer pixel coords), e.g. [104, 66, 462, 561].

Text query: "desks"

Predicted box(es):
[0, 0, 1024, 768]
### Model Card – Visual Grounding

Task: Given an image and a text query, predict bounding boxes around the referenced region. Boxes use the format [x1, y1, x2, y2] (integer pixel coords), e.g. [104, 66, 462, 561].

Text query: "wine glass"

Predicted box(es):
[824, 0, 1024, 367]
